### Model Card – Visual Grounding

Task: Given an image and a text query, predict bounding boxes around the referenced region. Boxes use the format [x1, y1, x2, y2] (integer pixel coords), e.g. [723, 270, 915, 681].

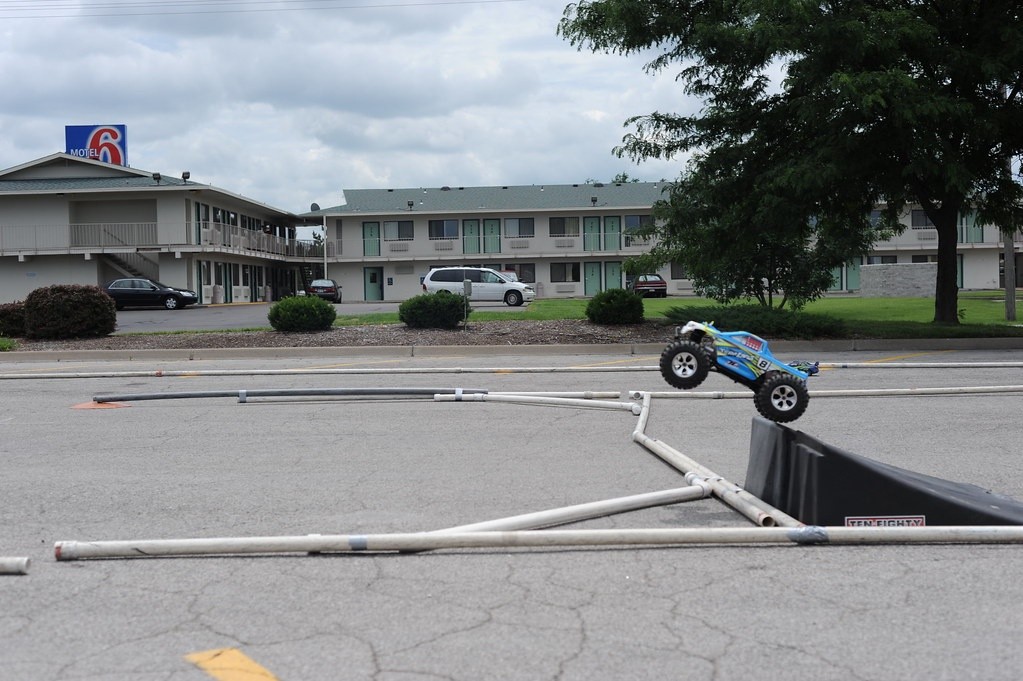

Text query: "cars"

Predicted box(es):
[631, 273, 668, 298]
[309, 279, 343, 304]
[100, 276, 198, 311]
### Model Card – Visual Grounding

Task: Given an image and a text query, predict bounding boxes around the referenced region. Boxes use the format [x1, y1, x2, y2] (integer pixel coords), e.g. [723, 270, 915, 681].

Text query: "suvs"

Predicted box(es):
[660, 320, 820, 425]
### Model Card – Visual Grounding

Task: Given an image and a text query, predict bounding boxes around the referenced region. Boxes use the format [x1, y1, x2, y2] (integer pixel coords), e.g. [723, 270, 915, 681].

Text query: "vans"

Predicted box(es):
[422, 266, 535, 307]
